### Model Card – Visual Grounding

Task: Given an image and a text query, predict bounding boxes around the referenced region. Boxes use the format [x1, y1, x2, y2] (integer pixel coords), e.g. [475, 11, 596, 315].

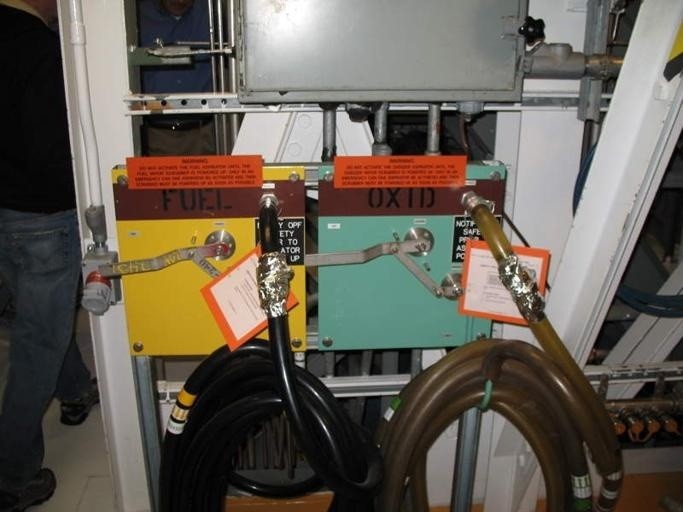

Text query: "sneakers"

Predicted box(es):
[0, 467, 57, 512]
[59, 375, 101, 426]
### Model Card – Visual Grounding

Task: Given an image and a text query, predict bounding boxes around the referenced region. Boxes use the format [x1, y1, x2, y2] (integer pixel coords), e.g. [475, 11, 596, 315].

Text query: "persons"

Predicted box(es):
[0, 1, 101, 510]
